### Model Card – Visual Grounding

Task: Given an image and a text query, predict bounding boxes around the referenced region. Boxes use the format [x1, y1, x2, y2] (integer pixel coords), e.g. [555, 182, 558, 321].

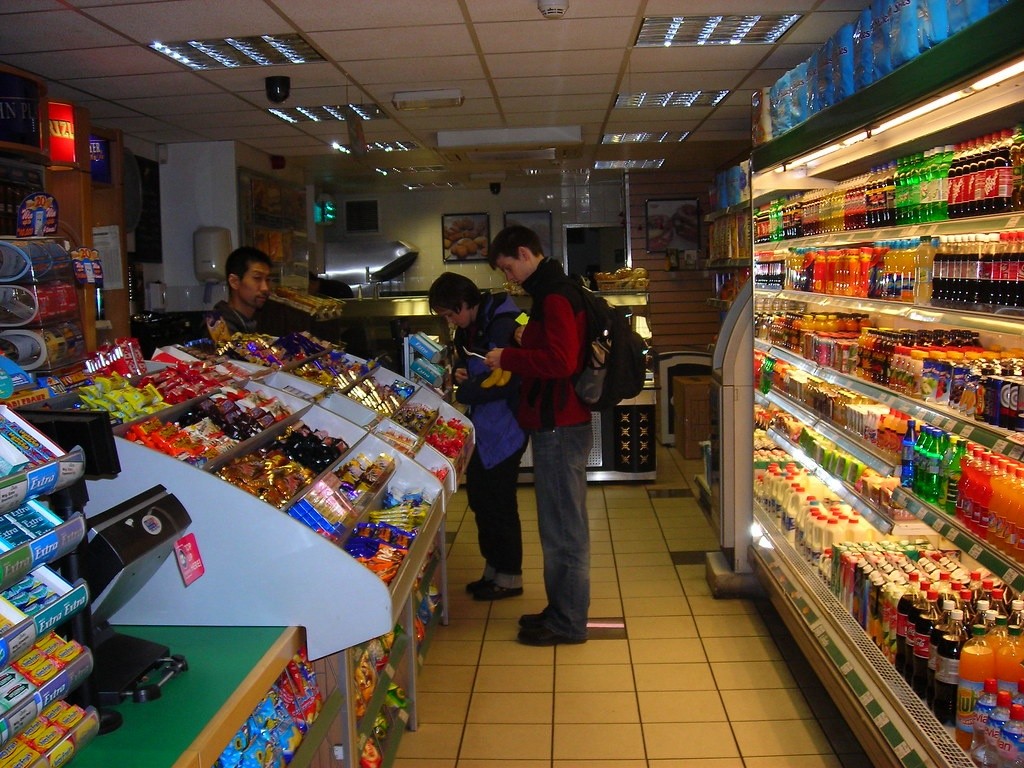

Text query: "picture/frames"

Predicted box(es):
[442, 211, 491, 262]
[645, 197, 701, 252]
[504, 210, 553, 259]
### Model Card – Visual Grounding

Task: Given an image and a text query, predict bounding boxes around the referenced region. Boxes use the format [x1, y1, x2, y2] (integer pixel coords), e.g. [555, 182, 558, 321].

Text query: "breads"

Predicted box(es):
[595, 267, 650, 291]
[445, 217, 489, 258]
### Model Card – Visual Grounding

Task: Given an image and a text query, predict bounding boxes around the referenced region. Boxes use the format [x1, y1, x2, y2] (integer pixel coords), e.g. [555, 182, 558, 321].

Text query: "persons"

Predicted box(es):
[429, 271, 530, 600]
[199, 245, 278, 339]
[308, 271, 364, 355]
[486, 224, 594, 646]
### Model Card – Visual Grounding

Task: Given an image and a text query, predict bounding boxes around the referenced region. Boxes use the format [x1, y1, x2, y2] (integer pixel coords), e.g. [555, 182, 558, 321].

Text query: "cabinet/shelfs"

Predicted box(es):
[1, 63, 476, 768]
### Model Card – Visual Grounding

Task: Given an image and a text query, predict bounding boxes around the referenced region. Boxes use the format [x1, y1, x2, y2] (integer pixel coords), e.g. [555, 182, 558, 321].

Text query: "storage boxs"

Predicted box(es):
[673, 376, 712, 458]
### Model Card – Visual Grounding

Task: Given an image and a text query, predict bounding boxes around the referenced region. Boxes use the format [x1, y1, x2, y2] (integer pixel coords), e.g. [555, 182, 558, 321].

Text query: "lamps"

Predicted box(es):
[392, 88, 466, 110]
[437, 125, 582, 149]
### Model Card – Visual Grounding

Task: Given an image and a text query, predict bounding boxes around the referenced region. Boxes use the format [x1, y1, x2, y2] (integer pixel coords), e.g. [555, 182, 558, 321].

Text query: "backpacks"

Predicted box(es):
[575, 293, 647, 410]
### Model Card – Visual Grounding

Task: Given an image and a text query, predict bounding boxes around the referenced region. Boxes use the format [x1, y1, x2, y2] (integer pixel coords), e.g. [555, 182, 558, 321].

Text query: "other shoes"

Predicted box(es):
[465, 575, 492, 594]
[517, 611, 547, 626]
[474, 582, 523, 601]
[518, 623, 586, 646]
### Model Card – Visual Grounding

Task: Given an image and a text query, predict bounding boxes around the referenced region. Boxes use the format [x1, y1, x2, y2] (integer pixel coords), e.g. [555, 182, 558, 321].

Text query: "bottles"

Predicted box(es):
[752, 232, 1024, 309]
[754, 311, 1024, 768]
[753, 122, 1024, 244]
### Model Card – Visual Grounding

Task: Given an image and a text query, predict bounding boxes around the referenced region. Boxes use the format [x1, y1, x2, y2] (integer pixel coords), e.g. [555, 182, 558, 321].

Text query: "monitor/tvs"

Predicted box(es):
[14, 405, 122, 481]
[57, 483, 192, 639]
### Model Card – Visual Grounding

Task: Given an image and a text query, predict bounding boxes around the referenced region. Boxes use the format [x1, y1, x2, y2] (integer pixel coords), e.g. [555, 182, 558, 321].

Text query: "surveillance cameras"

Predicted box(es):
[265, 75, 291, 103]
[490, 181, 501, 195]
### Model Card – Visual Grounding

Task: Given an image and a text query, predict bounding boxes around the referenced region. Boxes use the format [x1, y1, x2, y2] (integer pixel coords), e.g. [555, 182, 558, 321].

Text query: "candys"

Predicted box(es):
[65, 329, 470, 585]
